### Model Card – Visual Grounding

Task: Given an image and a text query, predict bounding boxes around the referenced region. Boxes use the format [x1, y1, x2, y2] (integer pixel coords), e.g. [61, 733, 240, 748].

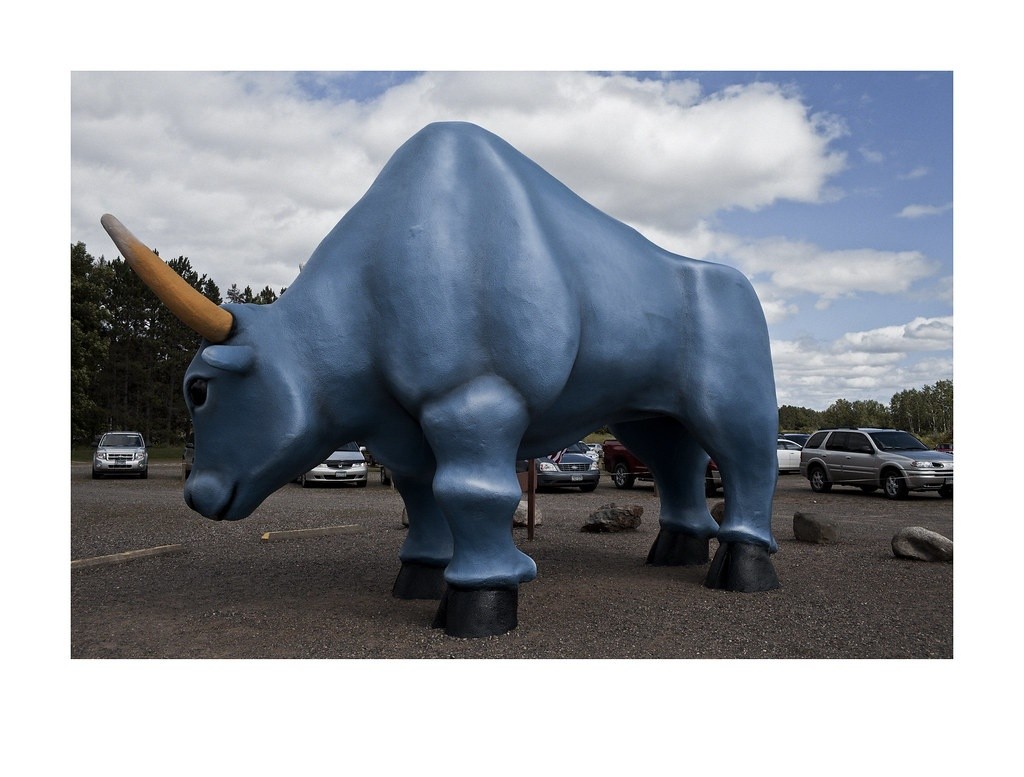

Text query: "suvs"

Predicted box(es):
[799, 427, 953, 499]
[92, 432, 148, 479]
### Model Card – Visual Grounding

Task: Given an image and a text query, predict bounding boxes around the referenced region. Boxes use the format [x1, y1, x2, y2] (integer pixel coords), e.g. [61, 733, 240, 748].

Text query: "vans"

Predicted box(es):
[783, 434, 811, 447]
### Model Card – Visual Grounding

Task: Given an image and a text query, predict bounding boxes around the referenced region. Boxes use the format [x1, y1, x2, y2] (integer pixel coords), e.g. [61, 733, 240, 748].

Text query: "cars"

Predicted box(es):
[776, 439, 804, 474]
[302, 439, 367, 487]
[534, 441, 600, 492]
[578, 440, 599, 465]
[933, 443, 953, 452]
[381, 462, 396, 483]
[586, 444, 604, 458]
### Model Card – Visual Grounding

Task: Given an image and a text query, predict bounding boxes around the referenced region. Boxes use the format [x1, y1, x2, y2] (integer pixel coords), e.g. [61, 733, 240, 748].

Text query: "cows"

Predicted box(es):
[100, 120, 782, 640]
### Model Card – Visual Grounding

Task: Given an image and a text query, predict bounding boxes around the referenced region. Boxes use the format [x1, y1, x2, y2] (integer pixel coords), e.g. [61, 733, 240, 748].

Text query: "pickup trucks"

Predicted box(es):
[603, 439, 723, 497]
